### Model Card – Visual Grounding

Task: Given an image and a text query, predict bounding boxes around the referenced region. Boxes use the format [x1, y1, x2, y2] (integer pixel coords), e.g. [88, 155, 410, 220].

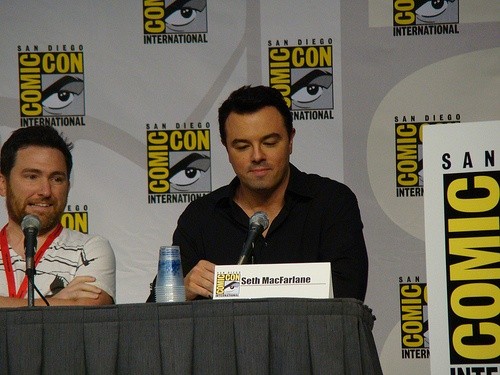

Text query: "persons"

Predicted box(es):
[0, 124, 117, 308]
[145, 83, 369, 303]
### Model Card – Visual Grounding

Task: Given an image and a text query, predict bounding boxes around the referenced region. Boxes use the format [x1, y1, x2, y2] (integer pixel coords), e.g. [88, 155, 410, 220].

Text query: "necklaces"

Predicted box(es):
[0, 221, 63, 299]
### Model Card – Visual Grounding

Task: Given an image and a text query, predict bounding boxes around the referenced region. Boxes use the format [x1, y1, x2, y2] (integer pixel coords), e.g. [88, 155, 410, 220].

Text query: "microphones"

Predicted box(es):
[237, 211, 270, 265]
[21, 214, 40, 276]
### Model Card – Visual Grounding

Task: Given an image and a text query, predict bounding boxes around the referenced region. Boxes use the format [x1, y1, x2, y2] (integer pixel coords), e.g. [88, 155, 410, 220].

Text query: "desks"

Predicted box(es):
[0, 299, 383, 375]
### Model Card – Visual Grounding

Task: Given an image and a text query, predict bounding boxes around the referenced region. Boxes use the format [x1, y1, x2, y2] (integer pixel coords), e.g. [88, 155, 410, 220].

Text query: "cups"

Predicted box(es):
[154, 246, 186, 303]
